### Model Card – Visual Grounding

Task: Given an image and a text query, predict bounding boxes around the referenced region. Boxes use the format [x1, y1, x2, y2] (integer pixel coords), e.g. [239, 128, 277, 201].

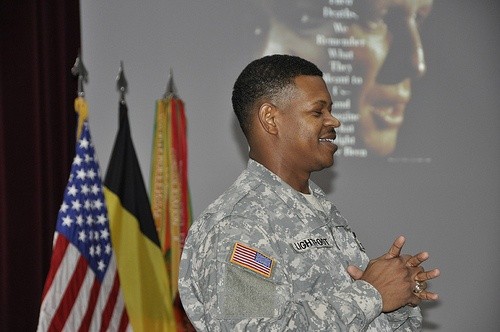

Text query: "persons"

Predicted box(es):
[178, 54, 442, 332]
[240, 1, 435, 160]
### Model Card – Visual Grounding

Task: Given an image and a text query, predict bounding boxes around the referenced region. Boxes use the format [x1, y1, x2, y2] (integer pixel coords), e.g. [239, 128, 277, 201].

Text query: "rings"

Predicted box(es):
[414, 280, 422, 294]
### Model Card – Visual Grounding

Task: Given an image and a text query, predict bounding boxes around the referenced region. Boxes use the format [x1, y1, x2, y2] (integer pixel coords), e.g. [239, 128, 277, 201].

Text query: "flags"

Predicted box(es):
[35, 92, 191, 332]
